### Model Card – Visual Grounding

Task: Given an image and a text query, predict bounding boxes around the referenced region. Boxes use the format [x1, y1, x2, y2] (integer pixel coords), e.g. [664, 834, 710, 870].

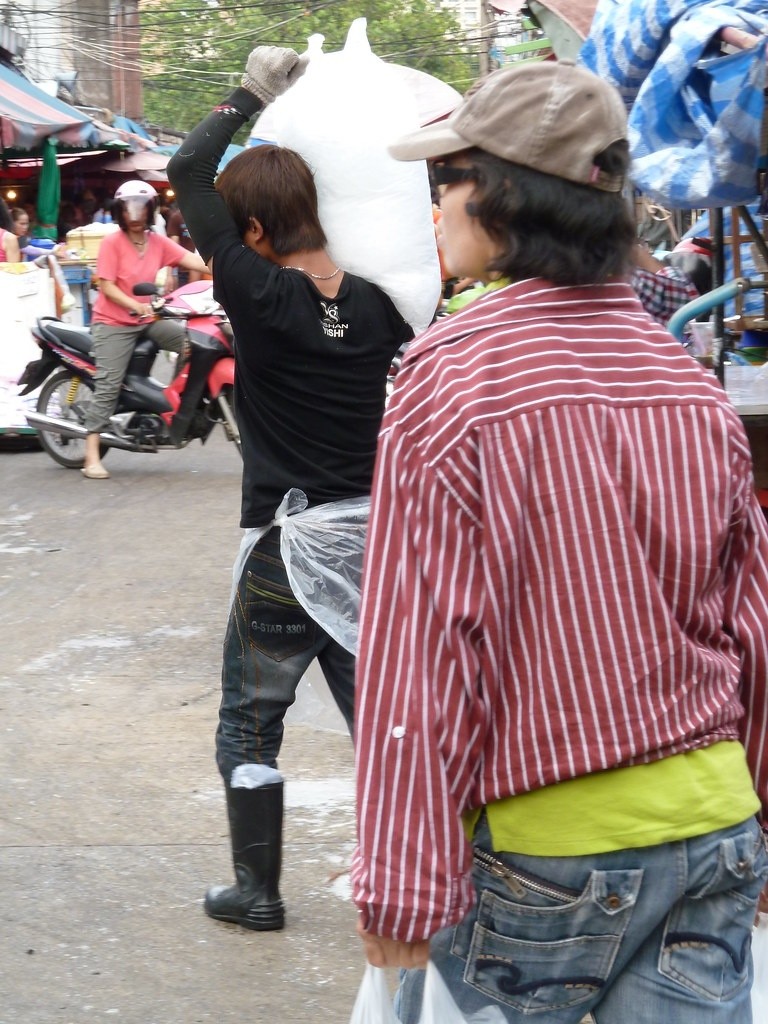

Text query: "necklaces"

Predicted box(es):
[133, 242, 144, 245]
[280, 265, 340, 280]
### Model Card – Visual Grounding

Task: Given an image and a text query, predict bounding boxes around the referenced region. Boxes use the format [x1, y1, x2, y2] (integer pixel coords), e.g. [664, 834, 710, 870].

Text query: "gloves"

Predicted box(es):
[241, 45, 311, 106]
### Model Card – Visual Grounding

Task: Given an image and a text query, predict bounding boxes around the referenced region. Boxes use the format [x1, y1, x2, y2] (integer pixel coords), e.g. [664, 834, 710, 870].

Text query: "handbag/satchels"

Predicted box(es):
[347, 952, 507, 1024]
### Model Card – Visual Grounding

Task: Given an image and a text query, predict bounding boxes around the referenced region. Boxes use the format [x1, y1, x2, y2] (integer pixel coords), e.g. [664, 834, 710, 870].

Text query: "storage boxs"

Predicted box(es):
[66, 231, 104, 259]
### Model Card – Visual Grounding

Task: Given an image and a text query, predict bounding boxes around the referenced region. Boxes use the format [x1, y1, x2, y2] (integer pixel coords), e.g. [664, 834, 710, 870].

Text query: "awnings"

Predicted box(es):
[0, 65, 95, 148]
[94, 123, 250, 180]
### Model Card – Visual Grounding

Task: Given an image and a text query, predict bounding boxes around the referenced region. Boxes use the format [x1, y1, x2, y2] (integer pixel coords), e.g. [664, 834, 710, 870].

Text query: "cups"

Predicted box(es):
[690, 321, 713, 355]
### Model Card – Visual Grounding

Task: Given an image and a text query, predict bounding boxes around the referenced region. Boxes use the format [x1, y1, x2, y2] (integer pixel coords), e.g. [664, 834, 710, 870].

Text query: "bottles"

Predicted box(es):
[104, 212, 112, 223]
[92, 208, 104, 223]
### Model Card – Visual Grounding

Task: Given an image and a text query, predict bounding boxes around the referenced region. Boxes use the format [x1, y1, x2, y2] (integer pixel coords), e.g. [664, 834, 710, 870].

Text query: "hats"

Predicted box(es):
[388, 58, 635, 195]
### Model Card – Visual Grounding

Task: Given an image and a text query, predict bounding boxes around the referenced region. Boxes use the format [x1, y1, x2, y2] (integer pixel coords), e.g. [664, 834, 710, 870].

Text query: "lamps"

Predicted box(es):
[55, 69, 79, 92]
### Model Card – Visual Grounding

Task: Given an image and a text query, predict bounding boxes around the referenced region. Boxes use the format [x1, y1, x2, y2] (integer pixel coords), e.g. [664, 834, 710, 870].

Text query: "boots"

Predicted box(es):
[204, 781, 285, 931]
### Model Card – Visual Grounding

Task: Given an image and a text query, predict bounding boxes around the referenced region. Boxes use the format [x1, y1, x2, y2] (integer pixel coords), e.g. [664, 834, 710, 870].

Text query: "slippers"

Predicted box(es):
[79, 461, 109, 478]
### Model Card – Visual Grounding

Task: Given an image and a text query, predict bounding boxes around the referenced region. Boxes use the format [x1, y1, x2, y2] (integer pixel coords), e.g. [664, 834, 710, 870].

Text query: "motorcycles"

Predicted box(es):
[14, 276, 243, 468]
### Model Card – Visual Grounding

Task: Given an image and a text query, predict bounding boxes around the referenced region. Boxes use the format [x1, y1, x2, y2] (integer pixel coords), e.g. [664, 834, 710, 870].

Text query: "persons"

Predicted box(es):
[82, 180, 211, 479]
[0, 197, 20, 263]
[151, 194, 201, 292]
[352, 59, 768, 1024]
[11, 208, 63, 262]
[631, 244, 700, 319]
[167, 46, 417, 933]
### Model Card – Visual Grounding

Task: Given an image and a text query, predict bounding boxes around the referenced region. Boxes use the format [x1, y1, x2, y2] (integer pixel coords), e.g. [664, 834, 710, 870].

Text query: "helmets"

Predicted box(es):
[112, 180, 161, 230]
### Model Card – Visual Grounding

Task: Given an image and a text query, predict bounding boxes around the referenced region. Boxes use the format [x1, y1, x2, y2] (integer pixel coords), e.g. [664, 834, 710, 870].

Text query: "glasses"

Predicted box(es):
[431, 157, 487, 187]
